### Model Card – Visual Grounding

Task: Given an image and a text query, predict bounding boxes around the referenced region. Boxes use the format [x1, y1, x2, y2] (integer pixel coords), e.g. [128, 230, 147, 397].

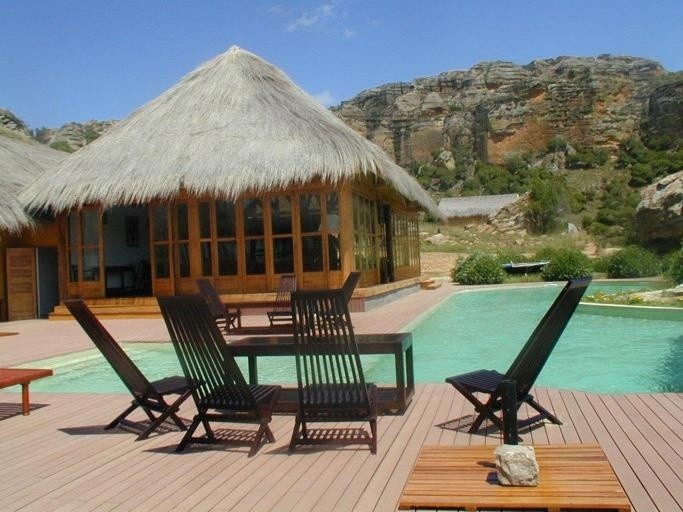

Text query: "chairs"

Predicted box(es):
[134, 260, 148, 294]
[72, 264, 95, 282]
[444, 275, 593, 445]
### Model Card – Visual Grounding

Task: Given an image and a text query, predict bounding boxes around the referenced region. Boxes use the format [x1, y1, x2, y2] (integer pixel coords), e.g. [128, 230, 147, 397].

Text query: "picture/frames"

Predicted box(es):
[125, 215, 140, 247]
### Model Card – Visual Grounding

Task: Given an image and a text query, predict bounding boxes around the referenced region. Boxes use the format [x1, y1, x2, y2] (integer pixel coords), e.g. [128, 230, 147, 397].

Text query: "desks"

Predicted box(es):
[398, 445, 631, 512]
[226, 333, 415, 419]
[0, 367, 53, 415]
[91, 265, 136, 297]
